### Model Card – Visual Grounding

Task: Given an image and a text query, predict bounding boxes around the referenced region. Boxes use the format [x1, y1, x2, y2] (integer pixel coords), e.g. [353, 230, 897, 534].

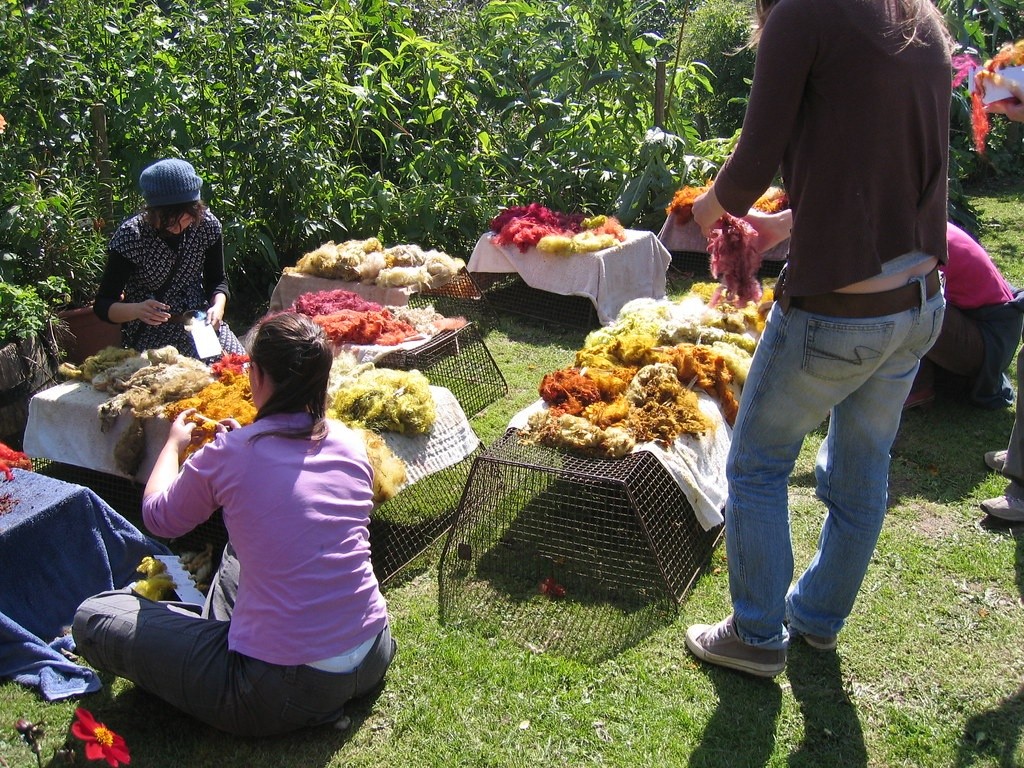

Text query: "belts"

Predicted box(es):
[791, 261, 940, 318]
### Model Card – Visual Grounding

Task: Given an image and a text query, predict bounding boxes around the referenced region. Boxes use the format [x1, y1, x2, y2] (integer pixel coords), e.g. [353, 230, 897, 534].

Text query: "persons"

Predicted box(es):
[93, 156, 248, 363]
[902, 79, 1024, 524]
[70, 310, 398, 738]
[685, 0, 950, 680]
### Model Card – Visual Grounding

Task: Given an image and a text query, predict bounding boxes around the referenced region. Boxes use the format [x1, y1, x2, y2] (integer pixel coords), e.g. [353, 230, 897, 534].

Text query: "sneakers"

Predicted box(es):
[981, 492, 1024, 520]
[686, 615, 786, 676]
[798, 626, 837, 651]
[983, 450, 1008, 473]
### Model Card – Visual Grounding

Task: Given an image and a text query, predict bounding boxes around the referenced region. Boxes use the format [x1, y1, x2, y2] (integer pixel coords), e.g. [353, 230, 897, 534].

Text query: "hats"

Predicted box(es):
[141, 157, 203, 207]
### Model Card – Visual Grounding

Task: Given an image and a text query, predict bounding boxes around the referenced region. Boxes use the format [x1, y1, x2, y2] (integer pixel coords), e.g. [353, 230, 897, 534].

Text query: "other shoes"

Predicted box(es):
[902, 381, 936, 412]
